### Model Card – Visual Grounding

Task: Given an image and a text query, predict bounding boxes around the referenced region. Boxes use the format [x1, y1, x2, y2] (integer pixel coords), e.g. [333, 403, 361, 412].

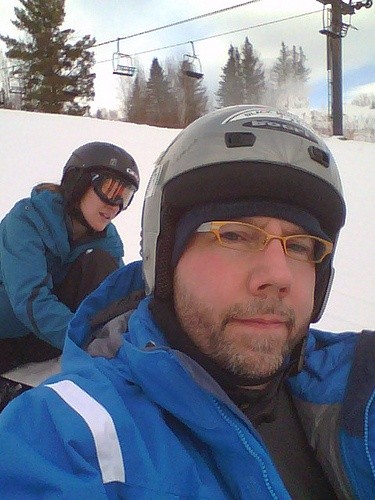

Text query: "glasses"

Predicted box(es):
[194, 221, 334, 263]
[83, 171, 137, 210]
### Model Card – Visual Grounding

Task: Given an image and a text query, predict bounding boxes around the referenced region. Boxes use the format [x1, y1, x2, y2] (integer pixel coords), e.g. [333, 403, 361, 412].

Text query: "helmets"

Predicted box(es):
[140, 105, 346, 322]
[63, 142, 140, 192]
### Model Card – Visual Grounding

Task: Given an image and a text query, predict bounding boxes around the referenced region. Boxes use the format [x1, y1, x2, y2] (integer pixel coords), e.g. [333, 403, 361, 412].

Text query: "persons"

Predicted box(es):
[0, 104, 375, 500]
